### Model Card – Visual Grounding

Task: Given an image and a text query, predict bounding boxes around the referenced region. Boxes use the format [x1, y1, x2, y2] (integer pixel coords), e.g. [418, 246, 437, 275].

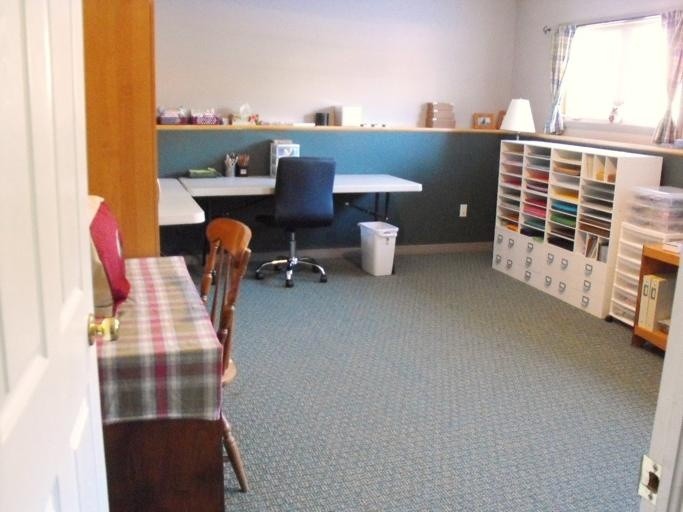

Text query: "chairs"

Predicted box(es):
[255, 157, 336, 287]
[200, 218, 252, 493]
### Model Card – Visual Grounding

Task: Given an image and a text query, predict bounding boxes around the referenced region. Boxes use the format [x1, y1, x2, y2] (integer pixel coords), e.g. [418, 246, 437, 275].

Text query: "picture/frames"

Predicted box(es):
[474, 112, 496, 129]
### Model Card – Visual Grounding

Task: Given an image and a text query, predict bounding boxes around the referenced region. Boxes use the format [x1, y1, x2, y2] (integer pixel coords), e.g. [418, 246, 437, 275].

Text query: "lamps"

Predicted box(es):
[500, 98, 535, 140]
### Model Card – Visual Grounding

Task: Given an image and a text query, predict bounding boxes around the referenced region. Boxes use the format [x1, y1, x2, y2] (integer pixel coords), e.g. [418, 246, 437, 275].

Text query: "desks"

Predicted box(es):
[157, 178, 206, 256]
[178, 174, 422, 285]
[95, 257, 224, 512]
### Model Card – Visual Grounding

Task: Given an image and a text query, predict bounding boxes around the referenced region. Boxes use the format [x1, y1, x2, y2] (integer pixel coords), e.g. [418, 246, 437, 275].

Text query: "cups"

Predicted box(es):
[224, 167, 235, 177]
[237, 166, 248, 176]
[673, 140, 682, 149]
[314, 111, 330, 127]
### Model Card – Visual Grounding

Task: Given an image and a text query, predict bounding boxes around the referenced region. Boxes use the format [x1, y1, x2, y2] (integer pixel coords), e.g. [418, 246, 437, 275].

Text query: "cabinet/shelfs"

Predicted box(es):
[606, 185, 683, 328]
[491, 139, 663, 320]
[631, 242, 680, 352]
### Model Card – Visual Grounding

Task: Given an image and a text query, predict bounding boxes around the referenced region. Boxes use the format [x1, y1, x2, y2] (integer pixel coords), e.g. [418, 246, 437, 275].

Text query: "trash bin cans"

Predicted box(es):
[358, 221, 400, 276]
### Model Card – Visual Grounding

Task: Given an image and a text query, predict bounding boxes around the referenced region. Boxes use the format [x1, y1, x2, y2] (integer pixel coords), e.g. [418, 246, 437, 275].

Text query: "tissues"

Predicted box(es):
[157, 109, 189, 125]
[192, 108, 222, 125]
[228, 102, 260, 126]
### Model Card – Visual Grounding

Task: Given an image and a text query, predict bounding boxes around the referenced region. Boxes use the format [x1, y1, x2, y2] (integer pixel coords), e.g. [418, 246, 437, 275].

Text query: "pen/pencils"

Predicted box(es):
[238, 153, 251, 165]
[223, 152, 238, 167]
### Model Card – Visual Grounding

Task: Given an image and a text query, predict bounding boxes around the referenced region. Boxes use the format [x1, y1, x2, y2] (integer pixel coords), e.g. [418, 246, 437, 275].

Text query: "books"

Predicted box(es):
[638, 272, 677, 333]
